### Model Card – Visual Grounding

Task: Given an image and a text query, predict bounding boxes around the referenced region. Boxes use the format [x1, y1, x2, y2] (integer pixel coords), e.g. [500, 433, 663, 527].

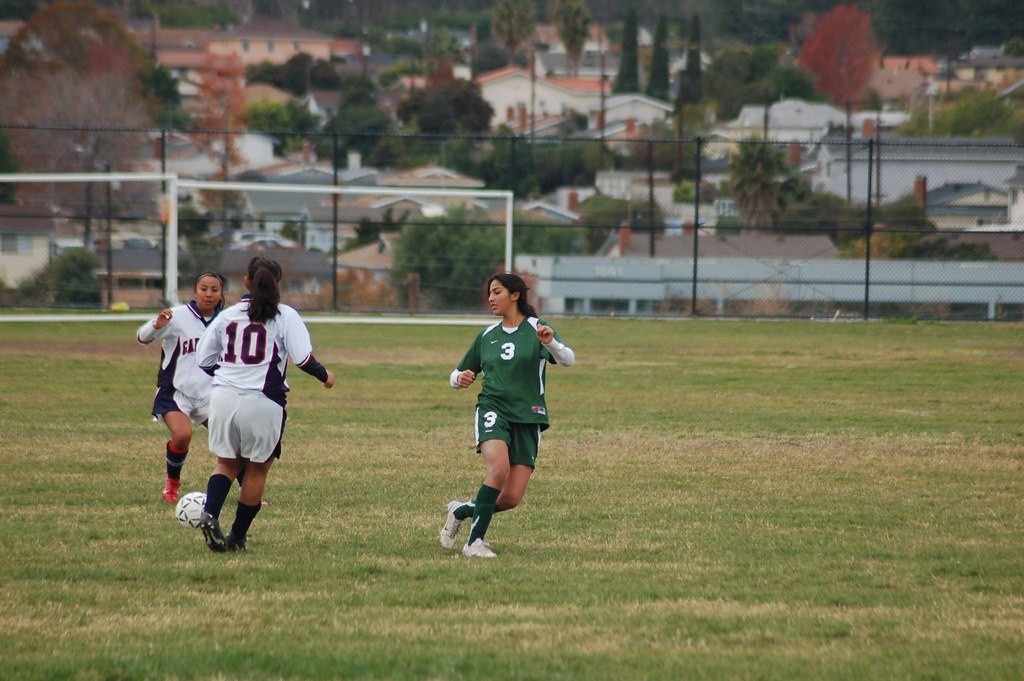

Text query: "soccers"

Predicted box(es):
[174, 490, 207, 530]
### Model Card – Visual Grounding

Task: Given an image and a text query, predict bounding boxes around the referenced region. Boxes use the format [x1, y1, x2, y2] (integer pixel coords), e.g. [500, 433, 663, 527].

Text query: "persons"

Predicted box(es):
[137, 272, 269, 507]
[196, 257, 334, 554]
[440, 273, 575, 559]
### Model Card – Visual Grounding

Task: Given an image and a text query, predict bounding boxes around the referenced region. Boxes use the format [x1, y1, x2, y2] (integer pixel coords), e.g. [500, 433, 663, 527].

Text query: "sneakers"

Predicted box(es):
[462, 538, 497, 558]
[440, 500, 467, 549]
[200, 512, 226, 553]
[164, 476, 181, 503]
[226, 531, 251, 552]
